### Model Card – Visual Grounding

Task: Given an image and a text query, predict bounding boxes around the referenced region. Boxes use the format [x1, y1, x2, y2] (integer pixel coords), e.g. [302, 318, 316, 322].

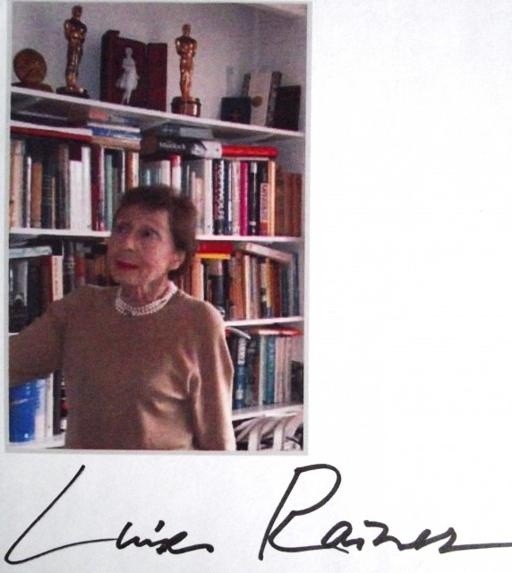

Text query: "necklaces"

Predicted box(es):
[114, 281, 177, 318]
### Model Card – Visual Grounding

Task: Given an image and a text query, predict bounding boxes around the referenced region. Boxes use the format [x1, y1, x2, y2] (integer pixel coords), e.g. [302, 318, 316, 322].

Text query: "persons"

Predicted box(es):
[8, 183, 237, 452]
[174, 23, 198, 98]
[116, 48, 138, 105]
[64, 5, 88, 88]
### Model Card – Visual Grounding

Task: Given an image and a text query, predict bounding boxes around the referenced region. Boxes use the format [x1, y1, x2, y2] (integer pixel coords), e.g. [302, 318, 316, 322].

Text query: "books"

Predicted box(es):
[9, 366, 65, 441]
[142, 122, 302, 238]
[204, 241, 300, 320]
[225, 326, 303, 410]
[8, 234, 109, 333]
[9, 98, 142, 232]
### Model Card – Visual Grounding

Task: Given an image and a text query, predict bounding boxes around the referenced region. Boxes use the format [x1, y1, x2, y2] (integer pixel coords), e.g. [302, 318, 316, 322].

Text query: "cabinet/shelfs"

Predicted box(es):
[4, 86, 306, 456]
